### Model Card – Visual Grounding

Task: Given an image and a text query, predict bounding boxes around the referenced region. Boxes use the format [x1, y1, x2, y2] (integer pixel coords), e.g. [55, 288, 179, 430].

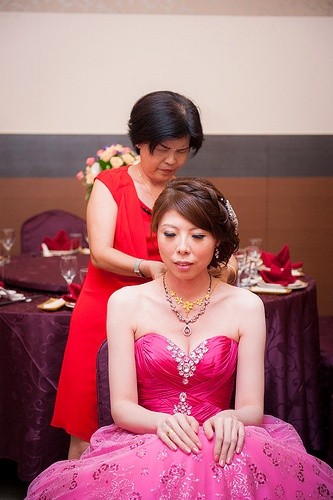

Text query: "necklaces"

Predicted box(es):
[164, 272, 219, 315]
[163, 269, 212, 337]
[137, 167, 156, 201]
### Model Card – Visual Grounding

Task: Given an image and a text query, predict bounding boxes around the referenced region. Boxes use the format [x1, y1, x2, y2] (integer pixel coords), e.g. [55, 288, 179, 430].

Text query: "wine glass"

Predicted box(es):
[0, 229, 16, 263]
[244, 247, 260, 289]
[59, 256, 78, 284]
[233, 248, 246, 288]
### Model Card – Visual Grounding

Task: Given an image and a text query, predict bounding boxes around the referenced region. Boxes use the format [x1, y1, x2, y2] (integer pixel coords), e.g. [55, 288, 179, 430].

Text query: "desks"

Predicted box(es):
[0, 268, 320, 484]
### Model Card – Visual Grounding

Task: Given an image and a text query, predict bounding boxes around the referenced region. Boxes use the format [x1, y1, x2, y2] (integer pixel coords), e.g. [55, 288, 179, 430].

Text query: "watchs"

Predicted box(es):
[134, 259, 145, 278]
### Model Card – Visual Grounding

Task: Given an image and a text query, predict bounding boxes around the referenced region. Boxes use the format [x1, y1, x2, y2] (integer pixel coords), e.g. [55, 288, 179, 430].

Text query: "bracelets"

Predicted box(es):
[229, 264, 236, 284]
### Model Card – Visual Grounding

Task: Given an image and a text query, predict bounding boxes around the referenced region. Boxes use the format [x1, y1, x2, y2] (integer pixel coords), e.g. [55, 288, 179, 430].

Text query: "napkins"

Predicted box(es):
[44, 228, 80, 251]
[59, 273, 87, 303]
[258, 259, 297, 286]
[260, 247, 302, 269]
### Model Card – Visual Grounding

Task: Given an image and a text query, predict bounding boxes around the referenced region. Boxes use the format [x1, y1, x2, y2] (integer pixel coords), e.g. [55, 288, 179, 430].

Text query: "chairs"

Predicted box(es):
[20, 209, 87, 253]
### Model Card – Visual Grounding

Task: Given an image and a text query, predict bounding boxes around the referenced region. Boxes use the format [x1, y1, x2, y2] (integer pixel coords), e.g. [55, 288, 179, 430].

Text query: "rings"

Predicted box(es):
[167, 429, 173, 435]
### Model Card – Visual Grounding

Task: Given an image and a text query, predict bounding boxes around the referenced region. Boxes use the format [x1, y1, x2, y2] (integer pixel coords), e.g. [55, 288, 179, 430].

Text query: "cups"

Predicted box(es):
[70, 234, 81, 258]
[84, 224, 89, 242]
[80, 268, 87, 281]
[249, 238, 263, 258]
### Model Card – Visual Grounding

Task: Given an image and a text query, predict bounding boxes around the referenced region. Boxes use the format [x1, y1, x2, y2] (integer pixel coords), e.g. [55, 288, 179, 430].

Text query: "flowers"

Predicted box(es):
[77, 144, 141, 197]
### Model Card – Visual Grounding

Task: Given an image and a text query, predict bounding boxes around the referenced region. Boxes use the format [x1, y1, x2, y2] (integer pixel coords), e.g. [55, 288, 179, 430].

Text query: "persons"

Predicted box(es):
[25, 176, 333, 500]
[51, 91, 237, 460]
